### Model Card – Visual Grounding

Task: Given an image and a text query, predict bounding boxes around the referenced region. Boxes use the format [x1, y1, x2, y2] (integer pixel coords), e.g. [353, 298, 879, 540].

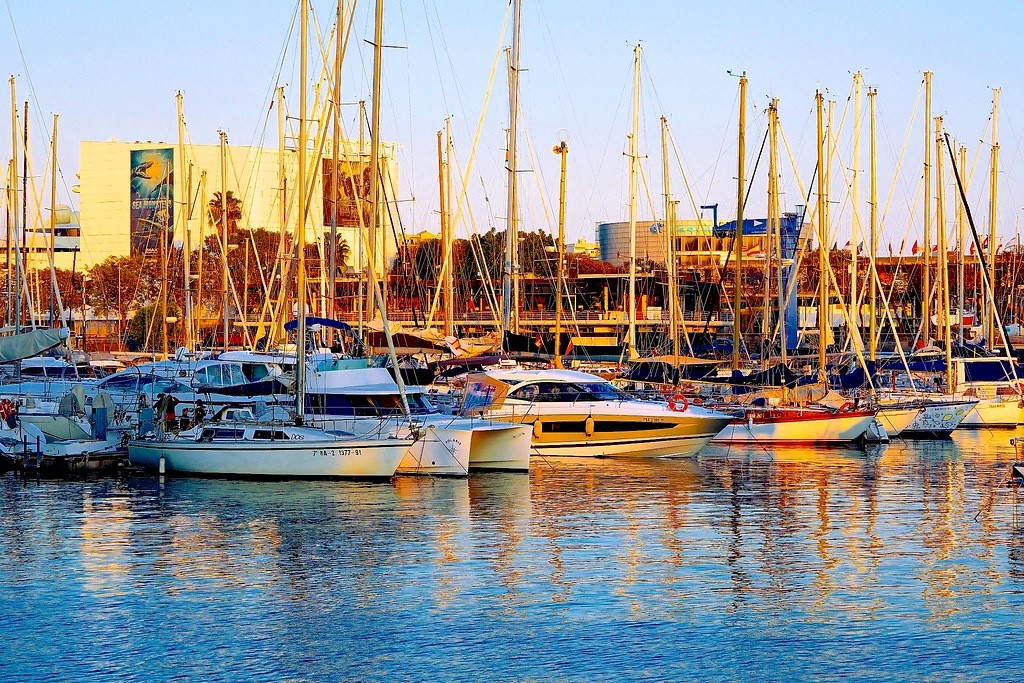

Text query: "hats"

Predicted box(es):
[141, 392, 146, 396]
[182, 408, 188, 412]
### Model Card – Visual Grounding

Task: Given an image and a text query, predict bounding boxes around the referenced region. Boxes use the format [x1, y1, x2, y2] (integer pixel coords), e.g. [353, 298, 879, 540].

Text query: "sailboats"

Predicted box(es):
[0, 0, 536, 485]
[615, 45, 1024, 443]
[468, 142, 735, 459]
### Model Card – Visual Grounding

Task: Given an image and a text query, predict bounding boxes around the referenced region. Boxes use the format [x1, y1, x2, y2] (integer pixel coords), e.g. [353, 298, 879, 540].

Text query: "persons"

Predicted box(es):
[152, 392, 206, 432]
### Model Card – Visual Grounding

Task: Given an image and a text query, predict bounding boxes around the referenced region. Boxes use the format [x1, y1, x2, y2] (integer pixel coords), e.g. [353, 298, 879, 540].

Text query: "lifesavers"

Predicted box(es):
[1, 398, 17, 424]
[839, 401, 859, 415]
[668, 393, 690, 414]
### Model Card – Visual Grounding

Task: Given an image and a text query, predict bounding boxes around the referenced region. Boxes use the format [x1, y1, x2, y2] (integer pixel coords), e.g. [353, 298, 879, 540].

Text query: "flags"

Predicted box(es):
[856, 241, 863, 254]
[970, 242, 974, 255]
[533, 336, 544, 348]
[565, 342, 573, 356]
[654, 345, 664, 355]
[983, 237, 988, 248]
[747, 242, 760, 257]
[912, 240, 919, 254]
